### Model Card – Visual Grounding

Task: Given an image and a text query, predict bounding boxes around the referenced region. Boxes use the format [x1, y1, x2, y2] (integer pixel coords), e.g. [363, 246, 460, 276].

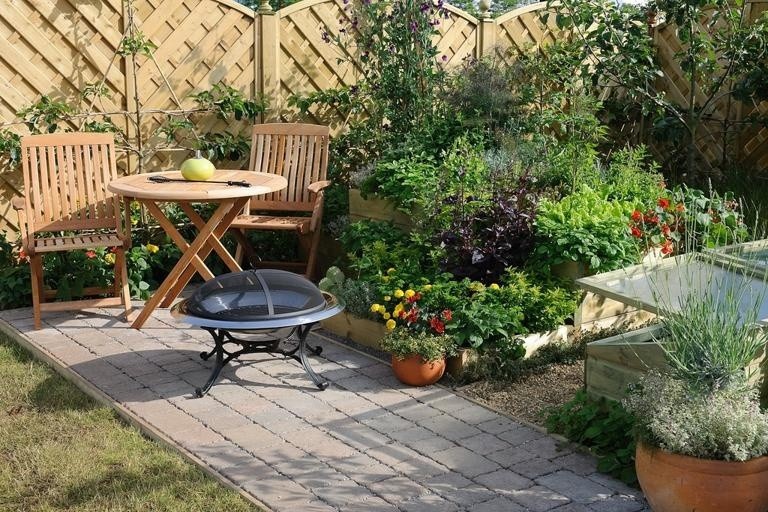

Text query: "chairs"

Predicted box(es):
[228, 120, 336, 280]
[15, 132, 135, 331]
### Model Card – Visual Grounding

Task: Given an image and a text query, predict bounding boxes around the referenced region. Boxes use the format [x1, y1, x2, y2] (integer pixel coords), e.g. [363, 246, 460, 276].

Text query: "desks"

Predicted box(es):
[107, 166, 288, 330]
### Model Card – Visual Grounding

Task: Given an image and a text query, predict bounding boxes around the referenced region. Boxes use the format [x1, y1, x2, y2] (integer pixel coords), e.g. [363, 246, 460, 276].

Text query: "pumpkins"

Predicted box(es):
[180, 150, 215, 181]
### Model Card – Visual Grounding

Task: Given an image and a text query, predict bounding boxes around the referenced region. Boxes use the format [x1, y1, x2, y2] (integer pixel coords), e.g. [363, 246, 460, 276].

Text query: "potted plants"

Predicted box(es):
[620, 373, 768, 509]
[377, 325, 452, 387]
